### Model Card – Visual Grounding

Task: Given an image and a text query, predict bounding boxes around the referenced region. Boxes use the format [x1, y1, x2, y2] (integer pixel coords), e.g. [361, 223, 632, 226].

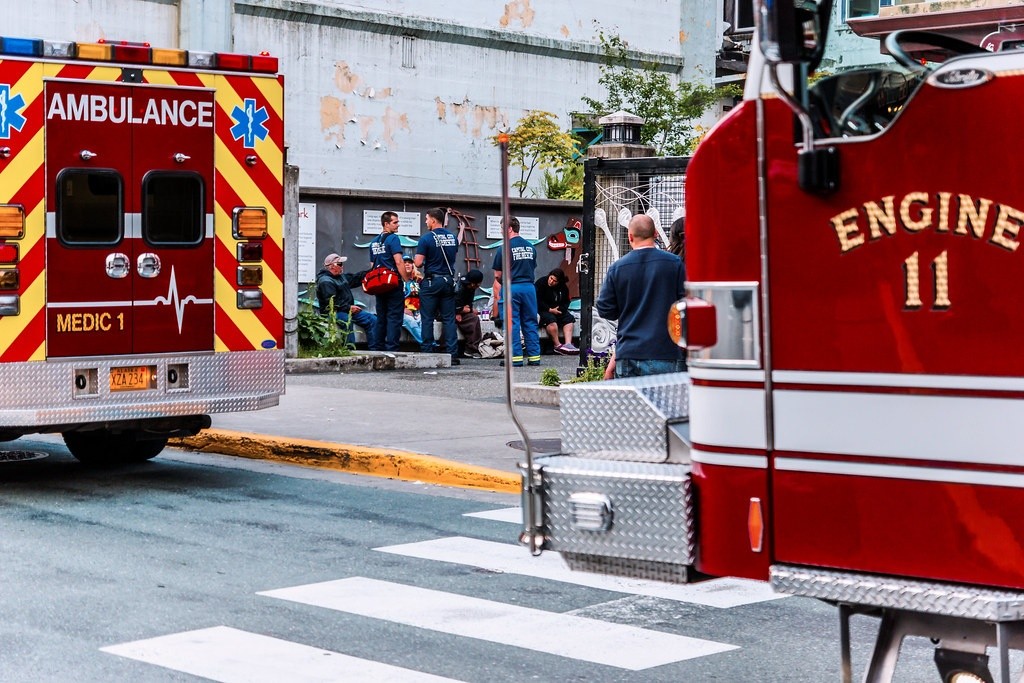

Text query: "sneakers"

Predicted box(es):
[560, 343, 580, 351]
[554, 343, 568, 356]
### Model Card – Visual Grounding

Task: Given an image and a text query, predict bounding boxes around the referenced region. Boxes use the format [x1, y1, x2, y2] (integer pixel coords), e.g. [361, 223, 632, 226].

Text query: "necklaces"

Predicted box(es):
[381, 231, 389, 234]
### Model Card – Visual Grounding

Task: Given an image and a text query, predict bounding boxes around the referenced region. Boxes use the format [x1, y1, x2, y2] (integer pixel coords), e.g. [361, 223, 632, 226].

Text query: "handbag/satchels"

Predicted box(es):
[360, 265, 399, 295]
[452, 276, 457, 287]
[478, 330, 505, 359]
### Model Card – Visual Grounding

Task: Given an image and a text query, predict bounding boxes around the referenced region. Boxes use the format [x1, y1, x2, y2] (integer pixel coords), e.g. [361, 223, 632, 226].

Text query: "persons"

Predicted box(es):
[491, 215, 541, 366]
[401, 255, 440, 348]
[316, 253, 377, 350]
[413, 208, 460, 365]
[436, 269, 483, 358]
[370, 211, 410, 351]
[534, 268, 580, 355]
[595, 214, 686, 381]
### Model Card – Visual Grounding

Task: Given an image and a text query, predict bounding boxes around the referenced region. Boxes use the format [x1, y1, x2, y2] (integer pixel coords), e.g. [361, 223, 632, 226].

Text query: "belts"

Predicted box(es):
[431, 273, 451, 277]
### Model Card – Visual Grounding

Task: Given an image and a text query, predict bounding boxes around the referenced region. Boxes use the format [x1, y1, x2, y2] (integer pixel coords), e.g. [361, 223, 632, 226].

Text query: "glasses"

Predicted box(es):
[334, 262, 342, 266]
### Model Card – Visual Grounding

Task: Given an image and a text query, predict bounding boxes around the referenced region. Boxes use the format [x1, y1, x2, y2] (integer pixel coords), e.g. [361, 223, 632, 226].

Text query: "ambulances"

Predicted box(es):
[0, 32, 289, 476]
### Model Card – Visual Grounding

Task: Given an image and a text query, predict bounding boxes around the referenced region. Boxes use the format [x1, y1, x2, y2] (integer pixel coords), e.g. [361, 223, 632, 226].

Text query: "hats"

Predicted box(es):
[459, 269, 483, 283]
[324, 253, 347, 266]
[402, 253, 414, 263]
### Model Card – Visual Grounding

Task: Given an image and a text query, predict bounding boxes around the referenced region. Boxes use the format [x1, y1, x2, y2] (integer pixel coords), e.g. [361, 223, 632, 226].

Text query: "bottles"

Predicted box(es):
[475, 303, 483, 321]
[482, 303, 490, 321]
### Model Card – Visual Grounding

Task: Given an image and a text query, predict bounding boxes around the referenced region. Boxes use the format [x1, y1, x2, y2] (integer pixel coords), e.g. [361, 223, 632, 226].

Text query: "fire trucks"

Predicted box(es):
[494, 1, 1024, 680]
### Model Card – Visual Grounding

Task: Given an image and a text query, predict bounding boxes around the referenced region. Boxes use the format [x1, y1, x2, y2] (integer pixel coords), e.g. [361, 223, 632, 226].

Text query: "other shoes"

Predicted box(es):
[527, 360, 541, 365]
[472, 353, 482, 358]
[451, 357, 460, 365]
[463, 350, 472, 356]
[432, 341, 440, 347]
[499, 361, 523, 367]
[414, 346, 432, 353]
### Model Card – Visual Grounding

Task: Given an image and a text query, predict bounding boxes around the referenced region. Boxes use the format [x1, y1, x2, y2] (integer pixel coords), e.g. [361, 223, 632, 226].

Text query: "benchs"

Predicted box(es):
[323, 319, 581, 343]
